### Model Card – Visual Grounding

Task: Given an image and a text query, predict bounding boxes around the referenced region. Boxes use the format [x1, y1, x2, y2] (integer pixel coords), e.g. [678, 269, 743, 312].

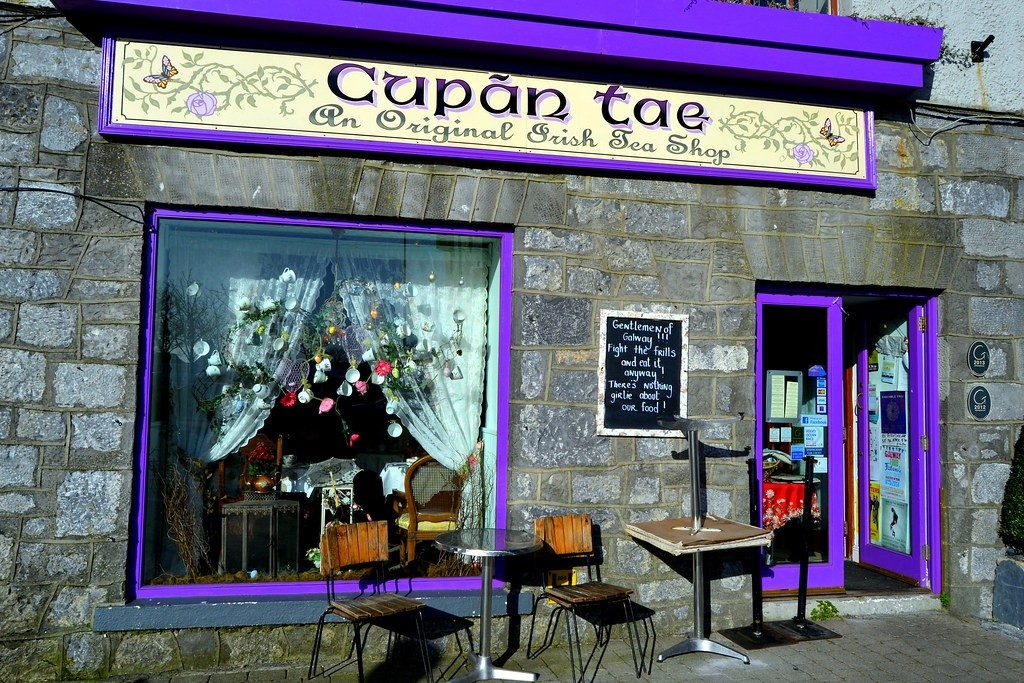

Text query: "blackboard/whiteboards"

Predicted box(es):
[595, 308, 691, 438]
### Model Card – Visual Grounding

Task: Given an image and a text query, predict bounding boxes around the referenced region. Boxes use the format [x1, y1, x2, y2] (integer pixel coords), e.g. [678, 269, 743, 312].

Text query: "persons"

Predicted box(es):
[870, 495, 879, 530]
[890, 508, 898, 537]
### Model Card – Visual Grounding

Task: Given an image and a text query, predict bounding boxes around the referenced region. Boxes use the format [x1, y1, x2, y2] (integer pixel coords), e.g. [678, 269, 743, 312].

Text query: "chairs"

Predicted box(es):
[526, 515, 640, 683]
[396, 457, 458, 569]
[308, 520, 433, 683]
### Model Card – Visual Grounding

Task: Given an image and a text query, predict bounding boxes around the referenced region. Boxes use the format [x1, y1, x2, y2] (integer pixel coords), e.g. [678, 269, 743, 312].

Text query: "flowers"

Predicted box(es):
[192, 277, 466, 442]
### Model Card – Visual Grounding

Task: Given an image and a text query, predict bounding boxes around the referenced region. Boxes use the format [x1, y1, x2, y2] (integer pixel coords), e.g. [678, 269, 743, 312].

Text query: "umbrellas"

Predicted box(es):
[298, 457, 364, 507]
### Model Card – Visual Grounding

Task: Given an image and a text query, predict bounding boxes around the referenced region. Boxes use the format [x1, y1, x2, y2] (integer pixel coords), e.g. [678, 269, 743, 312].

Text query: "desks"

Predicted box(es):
[434, 528, 544, 683]
[220, 499, 302, 577]
[762, 478, 820, 544]
